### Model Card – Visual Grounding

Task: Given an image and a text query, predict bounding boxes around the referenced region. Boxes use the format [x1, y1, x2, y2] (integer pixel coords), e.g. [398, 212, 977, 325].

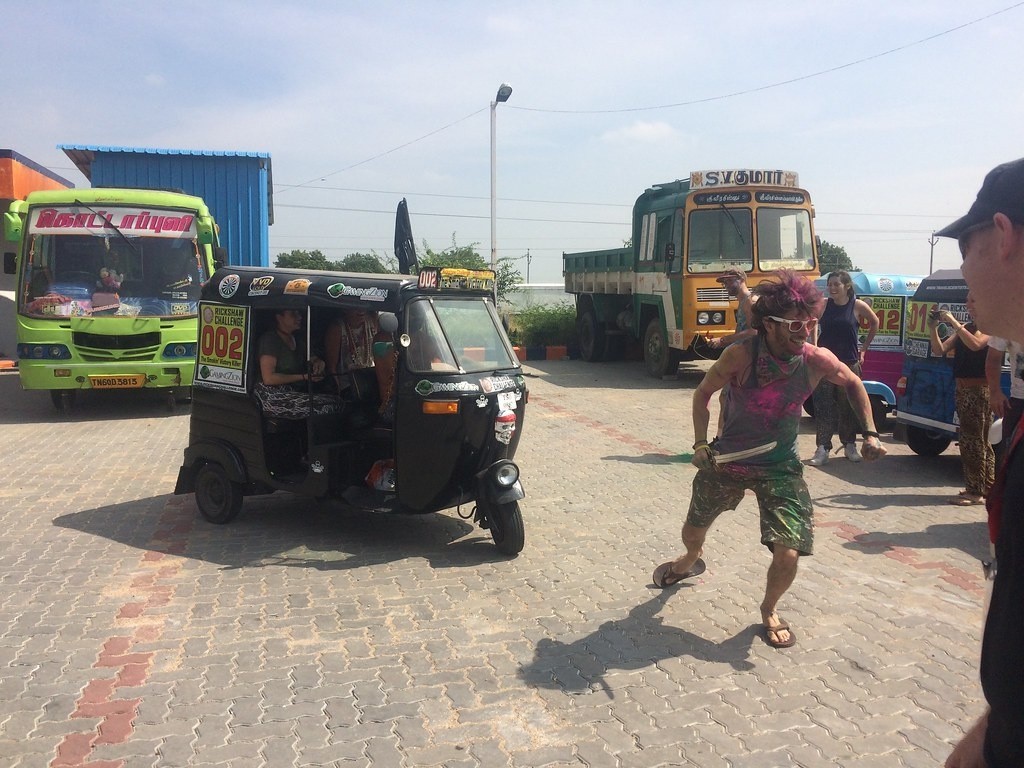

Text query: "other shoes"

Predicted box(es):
[691, 435, 719, 452]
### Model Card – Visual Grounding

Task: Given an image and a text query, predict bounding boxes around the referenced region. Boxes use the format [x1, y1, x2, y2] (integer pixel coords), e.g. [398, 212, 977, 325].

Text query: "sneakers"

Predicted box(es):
[844, 443, 863, 462]
[809, 445, 829, 466]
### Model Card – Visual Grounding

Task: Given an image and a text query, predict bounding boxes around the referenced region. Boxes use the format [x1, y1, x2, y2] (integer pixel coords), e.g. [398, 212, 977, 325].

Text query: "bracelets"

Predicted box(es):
[956, 326, 963, 333]
[693, 440, 707, 448]
[695, 446, 709, 453]
[721, 338, 724, 345]
[862, 431, 879, 438]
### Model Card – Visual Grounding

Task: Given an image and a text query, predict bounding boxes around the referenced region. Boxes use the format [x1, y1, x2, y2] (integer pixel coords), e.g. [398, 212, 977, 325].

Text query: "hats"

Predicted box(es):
[932, 157, 1024, 237]
[715, 267, 747, 282]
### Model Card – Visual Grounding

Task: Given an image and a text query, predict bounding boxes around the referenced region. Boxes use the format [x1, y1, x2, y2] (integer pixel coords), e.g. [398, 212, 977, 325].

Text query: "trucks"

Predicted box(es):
[558, 166, 821, 381]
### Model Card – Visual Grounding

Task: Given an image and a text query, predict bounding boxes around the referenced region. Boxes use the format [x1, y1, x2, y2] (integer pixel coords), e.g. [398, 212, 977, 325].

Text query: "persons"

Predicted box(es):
[705, 268, 758, 446]
[985, 337, 1024, 450]
[806, 270, 879, 466]
[256, 308, 346, 420]
[326, 307, 380, 393]
[928, 291, 995, 505]
[653, 268, 888, 648]
[931, 159, 1024, 767]
[372, 306, 482, 420]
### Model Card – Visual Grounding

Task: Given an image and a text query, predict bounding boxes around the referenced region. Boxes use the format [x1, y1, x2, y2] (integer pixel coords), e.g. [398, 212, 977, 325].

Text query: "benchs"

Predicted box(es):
[252, 349, 358, 473]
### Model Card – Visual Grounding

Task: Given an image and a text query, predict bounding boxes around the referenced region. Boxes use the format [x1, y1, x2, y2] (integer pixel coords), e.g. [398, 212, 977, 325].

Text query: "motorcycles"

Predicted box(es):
[889, 267, 1008, 458]
[806, 272, 930, 434]
[172, 265, 531, 557]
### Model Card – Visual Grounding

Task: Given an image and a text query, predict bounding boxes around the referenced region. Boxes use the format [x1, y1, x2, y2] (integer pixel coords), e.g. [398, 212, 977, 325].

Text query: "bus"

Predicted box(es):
[2, 186, 225, 405]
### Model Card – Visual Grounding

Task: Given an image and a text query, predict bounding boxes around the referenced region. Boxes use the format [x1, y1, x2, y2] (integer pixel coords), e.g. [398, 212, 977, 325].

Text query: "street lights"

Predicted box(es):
[488, 80, 512, 314]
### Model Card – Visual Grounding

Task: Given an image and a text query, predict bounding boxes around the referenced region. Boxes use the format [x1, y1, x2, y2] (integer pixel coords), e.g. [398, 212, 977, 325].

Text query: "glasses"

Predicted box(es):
[766, 314, 819, 332]
[960, 215, 1011, 260]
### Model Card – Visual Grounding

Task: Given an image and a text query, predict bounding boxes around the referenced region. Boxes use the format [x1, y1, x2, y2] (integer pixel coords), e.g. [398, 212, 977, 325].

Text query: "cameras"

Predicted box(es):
[931, 312, 942, 320]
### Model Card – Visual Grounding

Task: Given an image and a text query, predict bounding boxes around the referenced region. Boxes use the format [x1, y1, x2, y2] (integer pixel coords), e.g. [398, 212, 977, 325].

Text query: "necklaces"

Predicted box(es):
[344, 321, 373, 368]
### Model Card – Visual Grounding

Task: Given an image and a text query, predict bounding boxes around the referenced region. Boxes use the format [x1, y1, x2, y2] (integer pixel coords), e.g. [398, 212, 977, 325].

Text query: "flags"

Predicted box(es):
[394, 201, 416, 275]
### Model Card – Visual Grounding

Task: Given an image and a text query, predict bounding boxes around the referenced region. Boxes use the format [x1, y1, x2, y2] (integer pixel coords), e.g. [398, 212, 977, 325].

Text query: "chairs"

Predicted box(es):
[345, 367, 392, 442]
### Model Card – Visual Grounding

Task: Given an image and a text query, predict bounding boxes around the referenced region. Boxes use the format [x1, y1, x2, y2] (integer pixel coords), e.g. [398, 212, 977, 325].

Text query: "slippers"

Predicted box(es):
[951, 491, 993, 506]
[765, 617, 795, 648]
[651, 558, 710, 589]
